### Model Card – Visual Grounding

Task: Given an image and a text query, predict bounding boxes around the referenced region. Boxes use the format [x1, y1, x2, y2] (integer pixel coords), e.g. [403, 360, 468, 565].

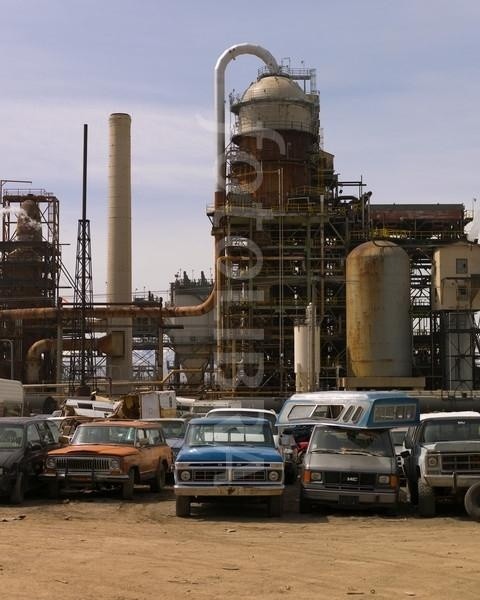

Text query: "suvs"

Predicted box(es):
[399, 409, 480, 520]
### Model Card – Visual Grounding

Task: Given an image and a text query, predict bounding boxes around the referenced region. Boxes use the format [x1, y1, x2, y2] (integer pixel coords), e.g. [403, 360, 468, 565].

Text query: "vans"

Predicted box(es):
[275, 390, 422, 431]
